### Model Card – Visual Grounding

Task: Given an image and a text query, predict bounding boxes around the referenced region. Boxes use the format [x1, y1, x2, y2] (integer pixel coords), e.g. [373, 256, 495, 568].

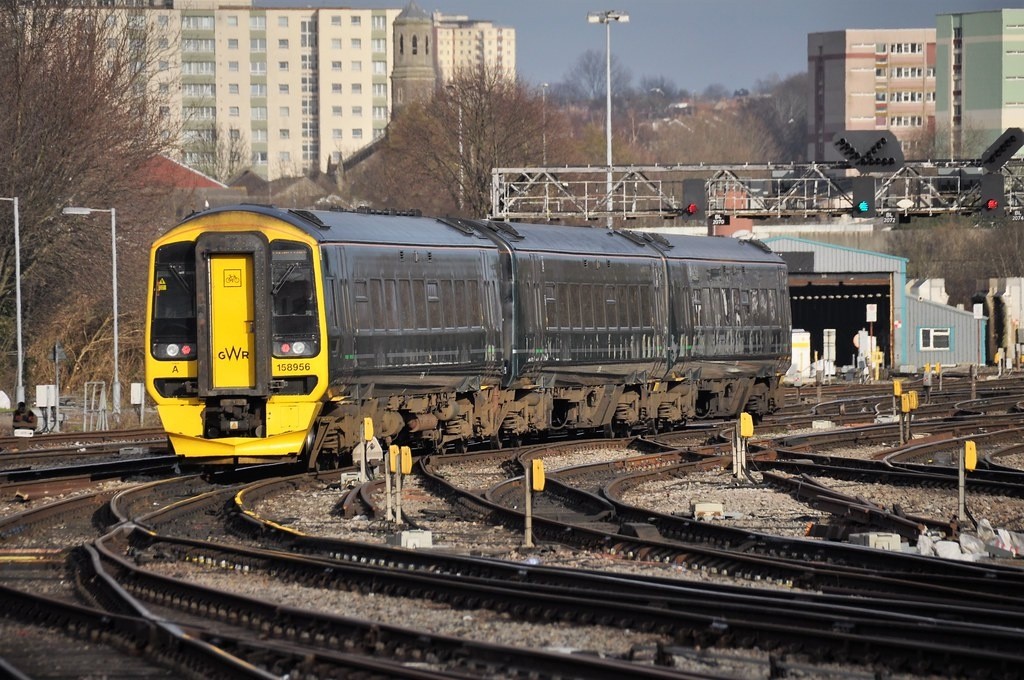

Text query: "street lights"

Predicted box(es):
[535, 83, 549, 168]
[445, 84, 465, 210]
[60, 207, 122, 425]
[585, 9, 630, 228]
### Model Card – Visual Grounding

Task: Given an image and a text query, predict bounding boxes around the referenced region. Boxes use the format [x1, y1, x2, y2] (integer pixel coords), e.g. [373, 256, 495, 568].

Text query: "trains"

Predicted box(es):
[143, 204, 791, 477]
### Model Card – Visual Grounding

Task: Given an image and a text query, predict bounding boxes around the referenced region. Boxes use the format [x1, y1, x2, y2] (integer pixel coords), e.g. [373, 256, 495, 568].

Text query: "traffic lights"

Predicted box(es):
[980, 173, 1004, 217]
[853, 175, 876, 218]
[681, 178, 705, 219]
